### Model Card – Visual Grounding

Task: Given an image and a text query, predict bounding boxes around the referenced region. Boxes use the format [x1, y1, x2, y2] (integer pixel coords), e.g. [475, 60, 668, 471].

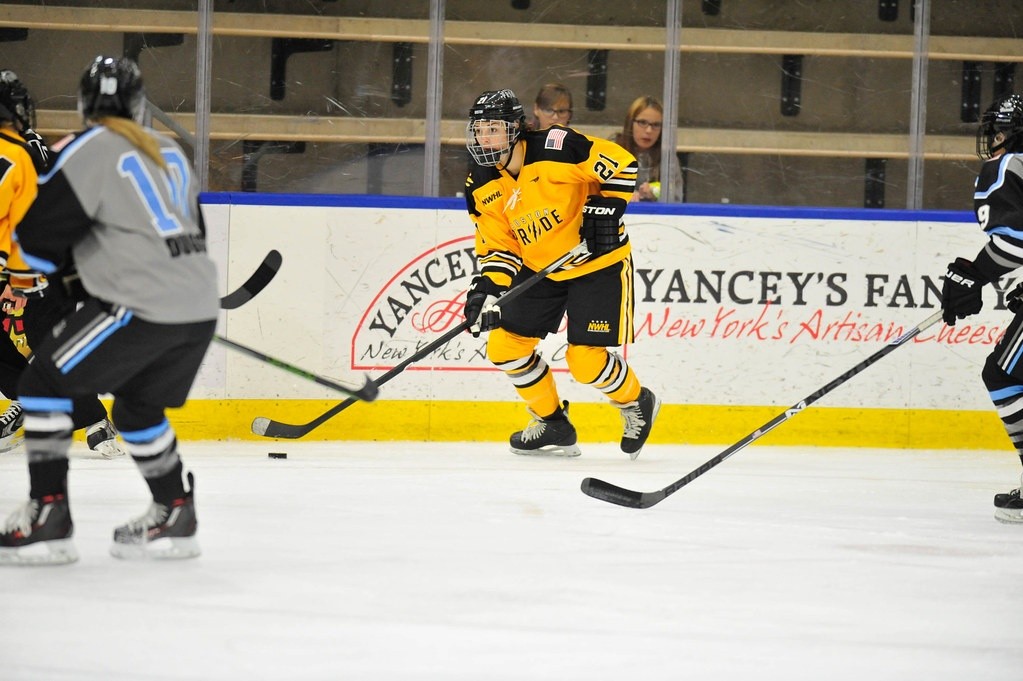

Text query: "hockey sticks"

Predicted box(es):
[219, 248, 285, 307]
[582, 312, 945, 507]
[211, 332, 379, 403]
[251, 242, 586, 439]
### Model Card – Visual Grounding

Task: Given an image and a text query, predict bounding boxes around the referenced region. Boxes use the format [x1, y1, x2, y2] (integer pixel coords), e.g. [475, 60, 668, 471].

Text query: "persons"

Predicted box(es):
[532, 84, 574, 130]
[0, 56, 222, 562]
[939, 93, 1023, 523]
[0, 69, 129, 459]
[605, 93, 684, 202]
[462, 87, 663, 460]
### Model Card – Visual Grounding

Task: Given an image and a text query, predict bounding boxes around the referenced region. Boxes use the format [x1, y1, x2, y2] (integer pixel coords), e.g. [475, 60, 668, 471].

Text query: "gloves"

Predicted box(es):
[578, 195, 627, 257]
[463, 274, 509, 338]
[940, 258, 983, 326]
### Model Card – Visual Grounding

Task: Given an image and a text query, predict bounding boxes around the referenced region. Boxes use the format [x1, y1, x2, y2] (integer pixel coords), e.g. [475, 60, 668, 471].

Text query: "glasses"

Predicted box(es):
[539, 107, 572, 119]
[634, 119, 662, 131]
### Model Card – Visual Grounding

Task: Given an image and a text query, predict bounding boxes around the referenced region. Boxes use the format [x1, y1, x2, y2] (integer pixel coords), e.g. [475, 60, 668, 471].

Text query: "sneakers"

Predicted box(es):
[993, 494, 1023, 523]
[609, 384, 661, 460]
[0, 487, 77, 566]
[86, 417, 125, 459]
[509, 401, 581, 456]
[110, 473, 201, 558]
[0, 399, 25, 452]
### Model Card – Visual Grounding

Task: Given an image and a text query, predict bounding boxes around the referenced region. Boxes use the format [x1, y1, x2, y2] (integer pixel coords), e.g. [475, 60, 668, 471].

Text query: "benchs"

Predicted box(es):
[1, 0, 1023, 207]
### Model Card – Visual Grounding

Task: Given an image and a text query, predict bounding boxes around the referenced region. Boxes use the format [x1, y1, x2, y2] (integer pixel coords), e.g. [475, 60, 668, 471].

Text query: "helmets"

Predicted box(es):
[976, 93, 1023, 161]
[0, 68, 37, 131]
[465, 88, 525, 167]
[79, 55, 142, 116]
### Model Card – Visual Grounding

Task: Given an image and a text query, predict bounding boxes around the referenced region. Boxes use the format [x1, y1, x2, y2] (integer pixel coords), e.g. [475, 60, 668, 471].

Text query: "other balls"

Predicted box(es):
[268, 452, 287, 459]
